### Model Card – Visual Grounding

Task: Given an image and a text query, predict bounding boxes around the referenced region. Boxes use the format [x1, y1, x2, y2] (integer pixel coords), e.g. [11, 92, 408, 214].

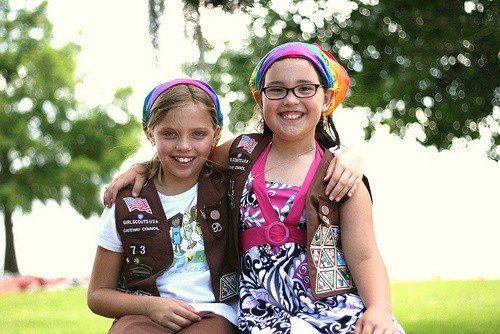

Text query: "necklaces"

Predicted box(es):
[245, 147, 314, 189]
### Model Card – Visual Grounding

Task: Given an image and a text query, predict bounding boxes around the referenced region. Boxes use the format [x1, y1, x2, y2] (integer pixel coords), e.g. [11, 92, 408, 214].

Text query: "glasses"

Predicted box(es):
[262, 83, 325, 100]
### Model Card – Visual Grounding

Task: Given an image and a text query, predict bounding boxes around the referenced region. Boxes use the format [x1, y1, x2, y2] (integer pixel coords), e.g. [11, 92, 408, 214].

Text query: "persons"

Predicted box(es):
[87, 79, 365, 334]
[102, 42, 406, 334]
